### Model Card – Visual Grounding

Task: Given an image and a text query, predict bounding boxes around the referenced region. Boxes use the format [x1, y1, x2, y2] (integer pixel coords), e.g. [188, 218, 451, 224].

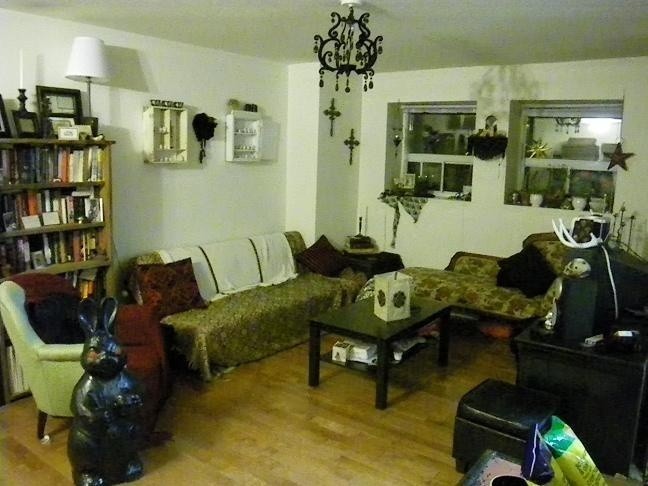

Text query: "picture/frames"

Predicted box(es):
[49, 113, 77, 139]
[36, 84, 84, 136]
[0, 93, 12, 137]
[10, 109, 41, 136]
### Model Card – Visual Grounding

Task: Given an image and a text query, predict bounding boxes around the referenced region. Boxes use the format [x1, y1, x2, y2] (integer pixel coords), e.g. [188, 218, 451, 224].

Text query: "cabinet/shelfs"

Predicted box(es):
[142, 106, 188, 166]
[226, 111, 262, 161]
[0, 138, 116, 409]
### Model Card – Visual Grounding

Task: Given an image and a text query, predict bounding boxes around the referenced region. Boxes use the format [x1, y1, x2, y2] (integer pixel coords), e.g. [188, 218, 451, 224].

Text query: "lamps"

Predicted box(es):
[310, 0, 385, 94]
[66, 36, 112, 137]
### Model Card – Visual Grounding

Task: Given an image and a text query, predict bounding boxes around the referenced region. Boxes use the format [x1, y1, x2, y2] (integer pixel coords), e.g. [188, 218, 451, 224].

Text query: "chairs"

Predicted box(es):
[0, 271, 169, 446]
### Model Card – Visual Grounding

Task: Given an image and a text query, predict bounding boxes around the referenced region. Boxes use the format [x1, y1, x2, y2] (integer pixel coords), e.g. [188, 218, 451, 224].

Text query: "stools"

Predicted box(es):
[450, 378, 576, 475]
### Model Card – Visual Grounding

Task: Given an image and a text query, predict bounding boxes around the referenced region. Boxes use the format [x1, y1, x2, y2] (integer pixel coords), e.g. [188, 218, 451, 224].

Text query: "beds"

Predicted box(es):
[354, 230, 588, 341]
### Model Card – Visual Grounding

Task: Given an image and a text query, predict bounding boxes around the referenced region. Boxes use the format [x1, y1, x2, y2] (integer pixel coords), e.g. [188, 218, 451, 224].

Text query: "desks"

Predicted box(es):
[509, 314, 646, 476]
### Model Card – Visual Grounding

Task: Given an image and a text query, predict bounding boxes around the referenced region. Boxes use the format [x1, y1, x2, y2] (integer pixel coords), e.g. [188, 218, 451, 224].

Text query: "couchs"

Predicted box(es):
[125, 230, 360, 382]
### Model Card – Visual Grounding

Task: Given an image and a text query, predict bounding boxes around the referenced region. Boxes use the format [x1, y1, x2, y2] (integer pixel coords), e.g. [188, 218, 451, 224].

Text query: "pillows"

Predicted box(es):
[497, 243, 555, 299]
[129, 256, 207, 319]
[494, 268, 510, 290]
[295, 234, 353, 278]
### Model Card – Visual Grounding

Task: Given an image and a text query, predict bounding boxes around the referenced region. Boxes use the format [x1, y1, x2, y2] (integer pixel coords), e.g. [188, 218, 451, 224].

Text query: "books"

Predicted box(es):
[3, 189, 105, 230]
[0, 145, 105, 184]
[2, 229, 100, 276]
[59, 268, 94, 297]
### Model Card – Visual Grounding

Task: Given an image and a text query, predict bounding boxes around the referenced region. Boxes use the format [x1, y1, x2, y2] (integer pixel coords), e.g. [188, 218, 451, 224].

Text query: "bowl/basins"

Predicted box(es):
[571, 197, 587, 210]
[529, 193, 544, 207]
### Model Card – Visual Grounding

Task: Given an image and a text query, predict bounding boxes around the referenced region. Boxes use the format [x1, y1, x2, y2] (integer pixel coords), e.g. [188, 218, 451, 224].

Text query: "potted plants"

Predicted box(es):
[572, 183, 590, 211]
[527, 186, 547, 208]
[588, 191, 608, 212]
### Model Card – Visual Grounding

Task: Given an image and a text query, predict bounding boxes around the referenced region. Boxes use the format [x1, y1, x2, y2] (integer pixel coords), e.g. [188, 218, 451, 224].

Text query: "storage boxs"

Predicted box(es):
[562, 137, 599, 162]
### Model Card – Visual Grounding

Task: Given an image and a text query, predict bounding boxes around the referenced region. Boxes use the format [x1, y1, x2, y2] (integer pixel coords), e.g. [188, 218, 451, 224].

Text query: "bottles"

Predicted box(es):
[512, 192, 520, 204]
